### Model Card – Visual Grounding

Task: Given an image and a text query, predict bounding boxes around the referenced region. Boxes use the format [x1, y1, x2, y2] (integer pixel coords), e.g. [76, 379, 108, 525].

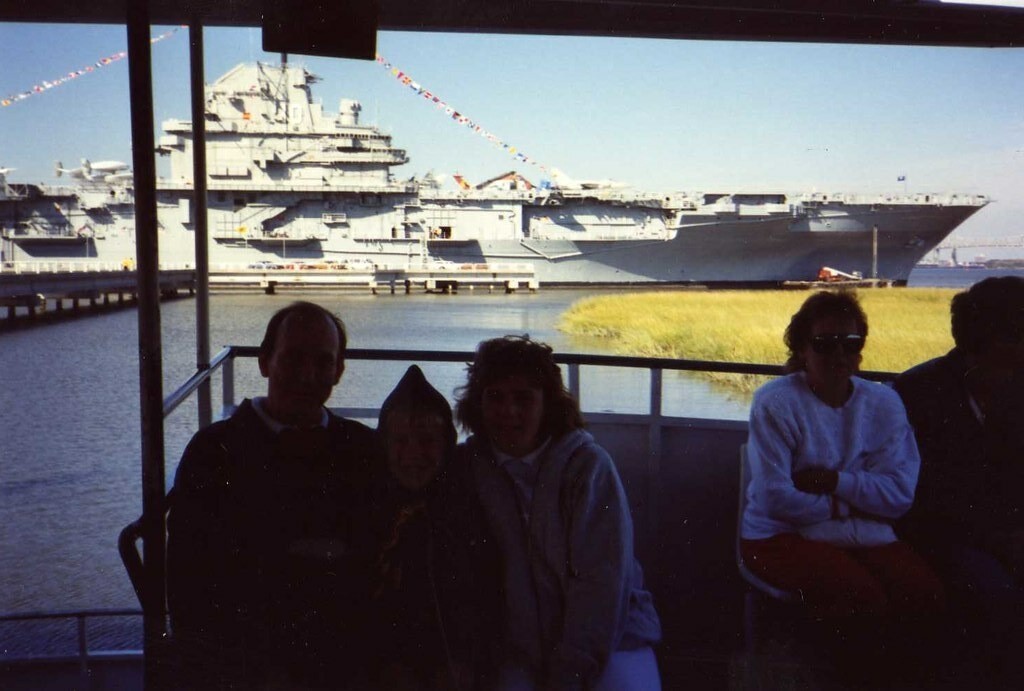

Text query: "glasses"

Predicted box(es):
[802, 333, 863, 356]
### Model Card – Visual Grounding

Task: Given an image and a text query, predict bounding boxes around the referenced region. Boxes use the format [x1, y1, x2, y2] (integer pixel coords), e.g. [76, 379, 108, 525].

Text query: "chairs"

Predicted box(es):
[734, 442, 801, 655]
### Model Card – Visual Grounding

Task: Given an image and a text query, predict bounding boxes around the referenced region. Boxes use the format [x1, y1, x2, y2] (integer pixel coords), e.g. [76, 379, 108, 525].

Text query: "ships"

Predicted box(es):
[1, 24, 994, 290]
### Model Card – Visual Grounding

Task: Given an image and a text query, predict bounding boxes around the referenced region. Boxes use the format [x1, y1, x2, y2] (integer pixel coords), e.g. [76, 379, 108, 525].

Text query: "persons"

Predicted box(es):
[157, 302, 663, 691]
[739, 279, 1024, 691]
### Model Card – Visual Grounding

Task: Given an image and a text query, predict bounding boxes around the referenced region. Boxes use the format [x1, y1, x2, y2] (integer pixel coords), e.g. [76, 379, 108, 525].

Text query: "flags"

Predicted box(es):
[455, 176, 470, 189]
[494, 140, 547, 172]
[447, 107, 493, 140]
[150, 25, 188, 44]
[0, 90, 33, 106]
[33, 66, 93, 94]
[95, 51, 128, 68]
[376, 57, 446, 108]
